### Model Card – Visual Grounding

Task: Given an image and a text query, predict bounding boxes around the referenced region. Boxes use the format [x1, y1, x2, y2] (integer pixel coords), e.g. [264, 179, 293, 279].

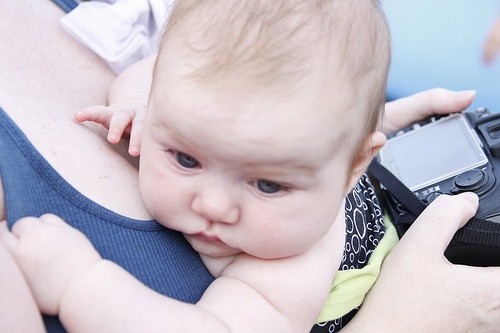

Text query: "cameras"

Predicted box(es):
[374, 107, 500, 233]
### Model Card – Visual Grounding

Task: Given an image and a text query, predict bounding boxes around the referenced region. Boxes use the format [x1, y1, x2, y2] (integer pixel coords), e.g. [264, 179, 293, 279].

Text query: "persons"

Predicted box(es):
[0, 1, 394, 333]
[0, 0, 500, 333]
